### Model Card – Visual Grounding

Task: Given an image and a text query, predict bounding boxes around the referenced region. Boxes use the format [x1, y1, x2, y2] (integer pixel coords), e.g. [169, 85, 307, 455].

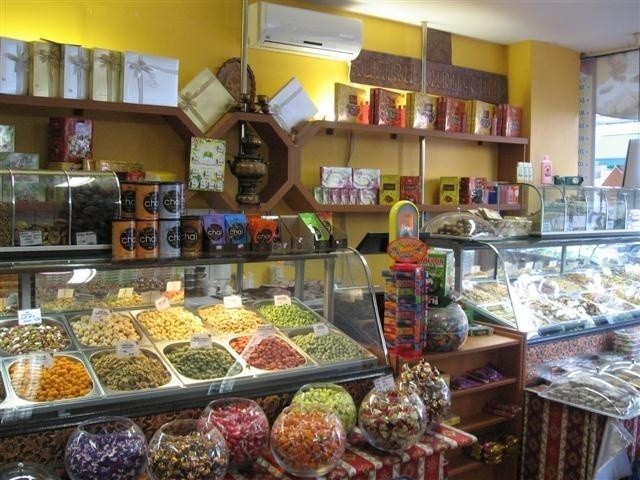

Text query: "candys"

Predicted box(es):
[379, 266, 429, 359]
[62, 359, 452, 480]
[426, 306, 468, 353]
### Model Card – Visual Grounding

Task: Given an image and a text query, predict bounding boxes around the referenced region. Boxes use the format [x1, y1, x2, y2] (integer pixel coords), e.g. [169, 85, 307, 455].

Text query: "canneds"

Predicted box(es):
[109, 180, 205, 261]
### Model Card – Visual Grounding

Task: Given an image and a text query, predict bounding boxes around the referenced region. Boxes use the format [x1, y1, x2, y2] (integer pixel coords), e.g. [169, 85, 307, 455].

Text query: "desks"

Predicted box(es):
[228, 417, 479, 480]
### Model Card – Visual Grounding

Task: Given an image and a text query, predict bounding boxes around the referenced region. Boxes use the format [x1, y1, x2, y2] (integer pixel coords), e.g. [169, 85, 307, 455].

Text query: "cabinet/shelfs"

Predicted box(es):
[197, 112, 295, 211]
[347, 321, 528, 479]
[281, 121, 529, 215]
[0, 93, 204, 206]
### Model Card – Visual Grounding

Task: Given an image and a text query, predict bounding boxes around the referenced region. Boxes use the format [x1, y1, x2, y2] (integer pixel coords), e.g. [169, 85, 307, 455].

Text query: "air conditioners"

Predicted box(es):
[241, 1, 364, 61]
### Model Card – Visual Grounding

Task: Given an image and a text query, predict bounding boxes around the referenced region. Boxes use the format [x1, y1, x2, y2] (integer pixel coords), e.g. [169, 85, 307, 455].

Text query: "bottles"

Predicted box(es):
[516, 162, 535, 183]
[541, 155, 552, 186]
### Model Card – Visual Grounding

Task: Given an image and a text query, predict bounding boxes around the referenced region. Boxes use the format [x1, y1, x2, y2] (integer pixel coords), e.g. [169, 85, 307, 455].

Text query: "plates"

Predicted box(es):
[218, 57, 256, 103]
